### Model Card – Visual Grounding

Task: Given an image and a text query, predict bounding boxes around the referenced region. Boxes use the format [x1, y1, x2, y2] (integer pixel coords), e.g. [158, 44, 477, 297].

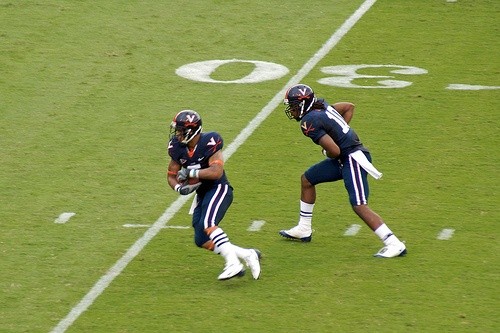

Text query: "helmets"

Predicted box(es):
[171, 109, 201, 144]
[284, 83, 315, 121]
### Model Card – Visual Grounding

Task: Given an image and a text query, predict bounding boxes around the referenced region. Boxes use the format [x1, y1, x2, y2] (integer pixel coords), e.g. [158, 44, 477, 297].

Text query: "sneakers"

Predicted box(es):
[373, 243, 406, 258]
[279, 227, 313, 242]
[218, 262, 246, 280]
[243, 249, 261, 280]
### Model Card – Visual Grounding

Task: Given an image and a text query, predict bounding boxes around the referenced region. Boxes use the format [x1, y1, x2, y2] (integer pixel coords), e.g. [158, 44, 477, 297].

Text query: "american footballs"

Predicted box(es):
[182, 179, 199, 185]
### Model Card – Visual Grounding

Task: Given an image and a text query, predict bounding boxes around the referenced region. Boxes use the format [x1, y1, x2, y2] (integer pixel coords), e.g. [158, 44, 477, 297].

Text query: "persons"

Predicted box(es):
[278, 83, 407, 258]
[166, 110, 262, 281]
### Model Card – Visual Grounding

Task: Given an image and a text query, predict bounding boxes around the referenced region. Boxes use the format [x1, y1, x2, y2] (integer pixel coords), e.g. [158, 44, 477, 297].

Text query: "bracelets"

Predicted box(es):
[174, 184, 180, 193]
[189, 169, 199, 179]
[323, 150, 327, 158]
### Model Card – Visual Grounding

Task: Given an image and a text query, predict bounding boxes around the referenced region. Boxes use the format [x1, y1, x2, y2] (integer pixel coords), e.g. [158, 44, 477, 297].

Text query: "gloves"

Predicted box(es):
[177, 168, 200, 182]
[175, 181, 202, 195]
